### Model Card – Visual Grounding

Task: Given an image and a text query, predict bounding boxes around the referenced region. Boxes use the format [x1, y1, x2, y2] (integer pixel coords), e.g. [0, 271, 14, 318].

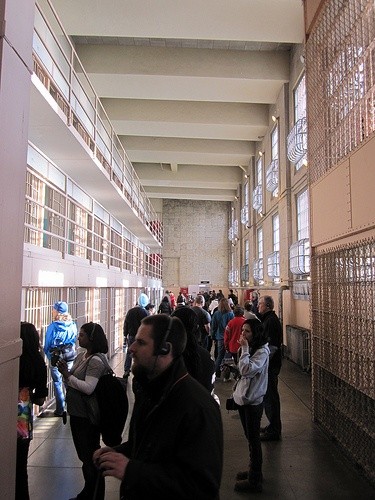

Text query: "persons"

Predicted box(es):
[15, 321, 48, 500]
[92, 314, 223, 500]
[257, 296, 283, 440]
[123, 293, 150, 379]
[44, 302, 78, 424]
[144, 290, 259, 382]
[56, 322, 111, 500]
[235, 318, 270, 495]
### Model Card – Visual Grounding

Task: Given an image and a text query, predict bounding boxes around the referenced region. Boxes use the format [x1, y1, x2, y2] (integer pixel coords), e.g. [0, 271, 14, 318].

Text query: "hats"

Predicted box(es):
[54, 302, 68, 313]
[138, 293, 149, 307]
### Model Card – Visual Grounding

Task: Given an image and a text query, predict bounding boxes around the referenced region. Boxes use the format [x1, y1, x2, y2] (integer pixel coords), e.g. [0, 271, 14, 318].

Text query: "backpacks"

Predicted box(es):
[84, 353, 128, 446]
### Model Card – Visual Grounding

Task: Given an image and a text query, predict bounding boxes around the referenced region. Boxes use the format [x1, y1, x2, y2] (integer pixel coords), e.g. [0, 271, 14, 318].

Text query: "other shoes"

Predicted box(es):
[260, 424, 270, 432]
[261, 432, 281, 441]
[224, 378, 232, 382]
[53, 412, 63, 417]
[215, 377, 224, 381]
[123, 370, 130, 378]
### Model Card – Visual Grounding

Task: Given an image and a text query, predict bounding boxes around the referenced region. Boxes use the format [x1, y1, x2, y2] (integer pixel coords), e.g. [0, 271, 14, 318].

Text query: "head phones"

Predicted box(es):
[157, 316, 173, 355]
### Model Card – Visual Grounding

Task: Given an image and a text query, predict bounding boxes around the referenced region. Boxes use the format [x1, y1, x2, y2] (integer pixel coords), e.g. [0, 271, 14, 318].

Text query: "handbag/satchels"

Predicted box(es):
[50, 343, 77, 366]
[226, 399, 238, 410]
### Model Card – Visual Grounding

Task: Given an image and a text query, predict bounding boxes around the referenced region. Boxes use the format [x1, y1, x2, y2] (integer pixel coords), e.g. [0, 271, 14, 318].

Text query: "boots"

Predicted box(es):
[234, 464, 264, 491]
[236, 470, 248, 480]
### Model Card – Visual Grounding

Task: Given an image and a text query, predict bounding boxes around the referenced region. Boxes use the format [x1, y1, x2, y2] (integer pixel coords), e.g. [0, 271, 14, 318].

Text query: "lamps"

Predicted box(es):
[235, 196, 239, 200]
[271, 115, 279, 122]
[244, 174, 250, 178]
[259, 212, 266, 217]
[258, 151, 265, 156]
